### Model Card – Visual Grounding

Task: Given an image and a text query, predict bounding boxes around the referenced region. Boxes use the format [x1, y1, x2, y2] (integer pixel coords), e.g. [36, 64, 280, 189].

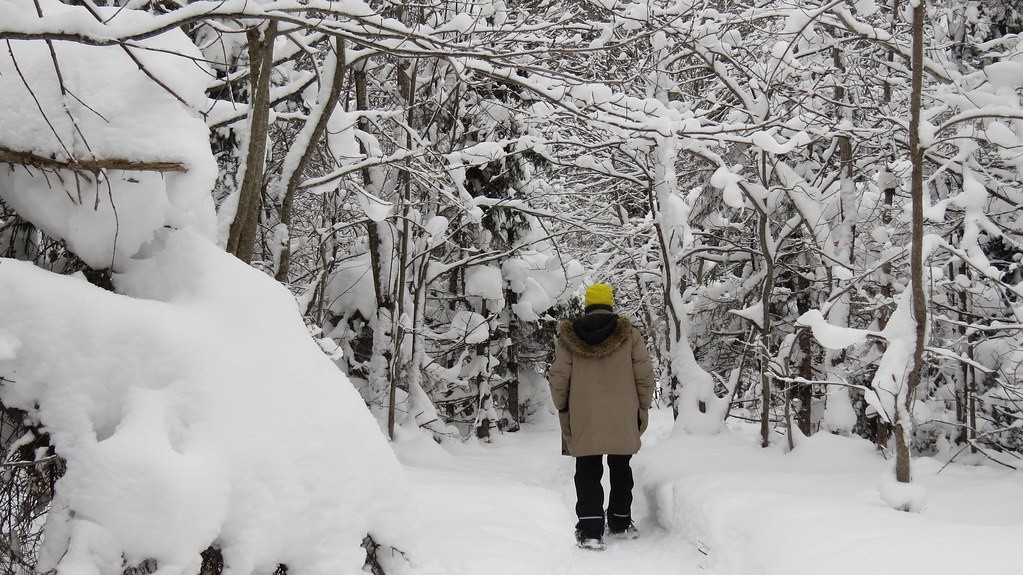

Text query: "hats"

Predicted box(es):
[585, 282, 614, 308]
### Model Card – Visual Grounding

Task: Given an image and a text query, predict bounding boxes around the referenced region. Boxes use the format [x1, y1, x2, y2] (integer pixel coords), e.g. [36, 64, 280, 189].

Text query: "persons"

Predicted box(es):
[548, 284, 655, 550]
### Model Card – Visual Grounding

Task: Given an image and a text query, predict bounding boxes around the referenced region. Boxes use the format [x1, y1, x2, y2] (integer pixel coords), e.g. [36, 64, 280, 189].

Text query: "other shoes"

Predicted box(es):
[607, 509, 631, 534]
[574, 528, 604, 548]
